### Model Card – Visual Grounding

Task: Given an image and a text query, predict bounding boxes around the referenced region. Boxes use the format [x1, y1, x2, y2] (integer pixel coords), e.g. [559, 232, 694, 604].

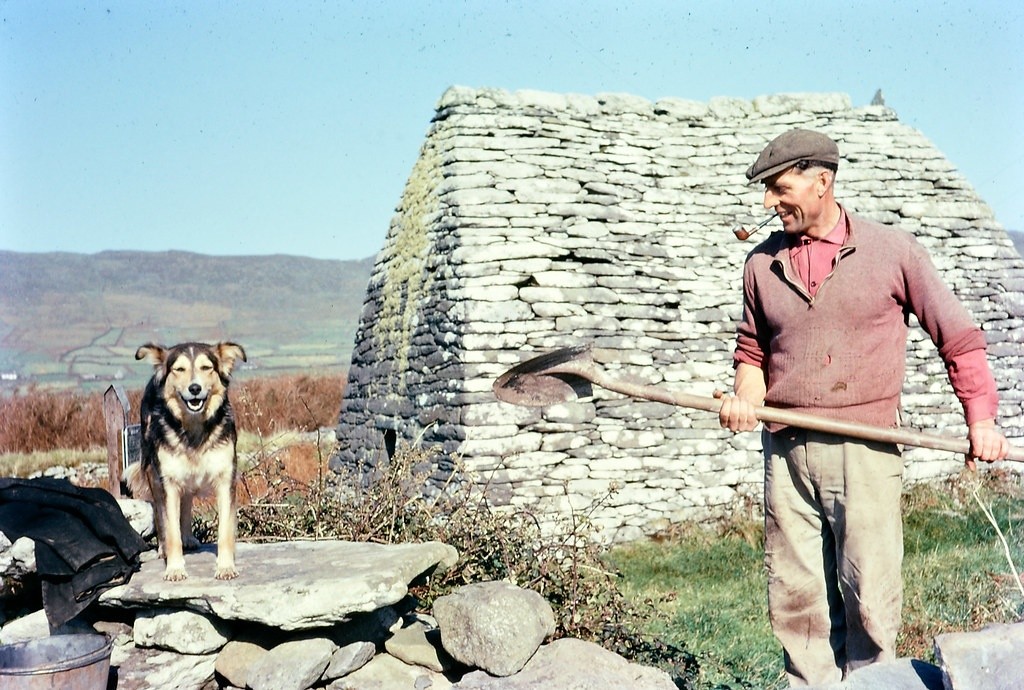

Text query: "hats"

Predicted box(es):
[746, 128, 839, 187]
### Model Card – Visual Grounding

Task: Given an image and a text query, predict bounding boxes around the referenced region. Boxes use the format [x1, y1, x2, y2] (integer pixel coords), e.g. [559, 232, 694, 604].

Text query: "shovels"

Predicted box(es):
[492, 341, 1024, 465]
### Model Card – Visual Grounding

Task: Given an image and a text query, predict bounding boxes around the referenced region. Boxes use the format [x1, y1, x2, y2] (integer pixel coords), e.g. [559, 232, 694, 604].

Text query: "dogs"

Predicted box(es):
[119, 339, 249, 582]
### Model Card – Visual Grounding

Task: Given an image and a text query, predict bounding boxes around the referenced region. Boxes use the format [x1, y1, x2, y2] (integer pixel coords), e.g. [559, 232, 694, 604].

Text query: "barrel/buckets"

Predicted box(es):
[0, 633, 113, 690]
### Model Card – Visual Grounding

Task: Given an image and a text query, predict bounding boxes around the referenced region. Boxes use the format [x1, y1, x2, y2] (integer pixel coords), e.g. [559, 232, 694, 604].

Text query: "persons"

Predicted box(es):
[711, 128, 1008, 688]
